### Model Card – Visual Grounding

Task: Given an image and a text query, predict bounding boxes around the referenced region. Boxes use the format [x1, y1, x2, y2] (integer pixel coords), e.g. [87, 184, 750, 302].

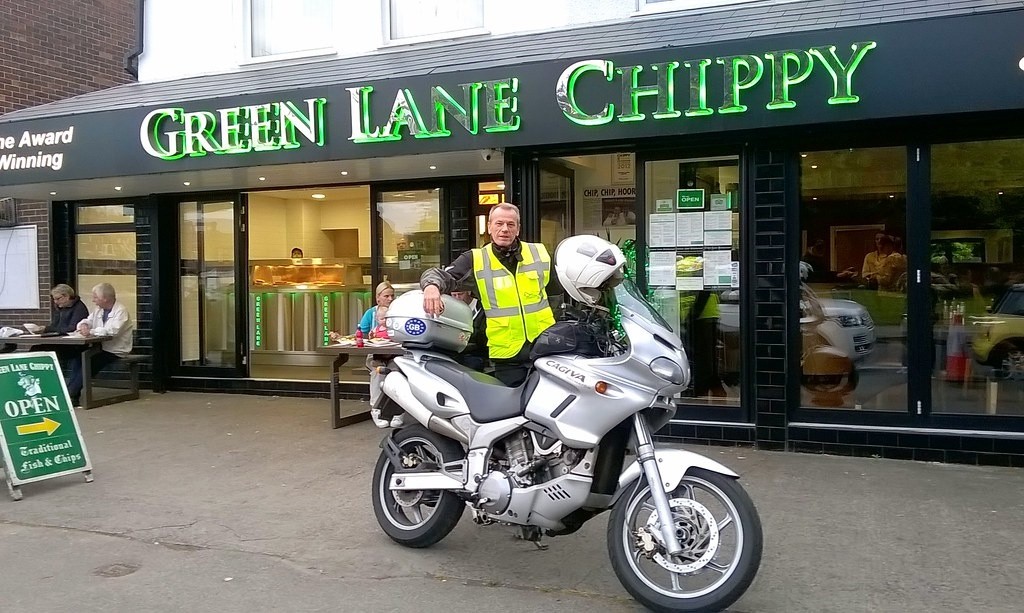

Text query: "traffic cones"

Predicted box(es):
[945, 313, 967, 381]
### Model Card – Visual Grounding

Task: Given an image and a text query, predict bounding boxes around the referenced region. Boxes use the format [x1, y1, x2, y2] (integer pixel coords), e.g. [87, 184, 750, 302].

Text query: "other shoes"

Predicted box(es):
[370, 408, 389, 429]
[390, 412, 405, 428]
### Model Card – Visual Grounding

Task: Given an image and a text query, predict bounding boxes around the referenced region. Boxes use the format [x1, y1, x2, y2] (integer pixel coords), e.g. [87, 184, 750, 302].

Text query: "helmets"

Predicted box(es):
[798, 260, 814, 285]
[553, 234, 627, 313]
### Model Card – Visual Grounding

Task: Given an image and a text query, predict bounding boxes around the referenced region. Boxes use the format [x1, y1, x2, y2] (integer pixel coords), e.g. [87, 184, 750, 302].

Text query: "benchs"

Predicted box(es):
[115, 354, 151, 398]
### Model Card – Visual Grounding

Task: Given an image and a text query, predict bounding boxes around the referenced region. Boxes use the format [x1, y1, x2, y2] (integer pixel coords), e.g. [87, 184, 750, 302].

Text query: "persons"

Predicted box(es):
[733, 274, 736, 279]
[328, 281, 405, 428]
[676, 255, 727, 403]
[861, 233, 907, 292]
[420, 203, 565, 387]
[801, 238, 855, 283]
[392, 239, 408, 261]
[368, 306, 400, 371]
[30, 284, 89, 363]
[603, 205, 636, 226]
[451, 290, 489, 372]
[955, 264, 1024, 298]
[66, 282, 133, 408]
[291, 248, 303, 258]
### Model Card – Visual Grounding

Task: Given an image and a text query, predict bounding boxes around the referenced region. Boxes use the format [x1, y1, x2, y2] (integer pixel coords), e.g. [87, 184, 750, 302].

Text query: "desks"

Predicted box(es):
[934, 316, 989, 339]
[315, 339, 405, 430]
[0, 336, 113, 410]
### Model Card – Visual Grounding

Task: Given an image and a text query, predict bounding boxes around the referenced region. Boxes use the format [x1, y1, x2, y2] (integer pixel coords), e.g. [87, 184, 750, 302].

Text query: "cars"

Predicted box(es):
[972, 278, 1024, 378]
[816, 296, 876, 362]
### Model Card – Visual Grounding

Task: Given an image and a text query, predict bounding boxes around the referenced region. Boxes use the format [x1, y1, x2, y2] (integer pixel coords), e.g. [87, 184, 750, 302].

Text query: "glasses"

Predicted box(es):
[52, 295, 64, 303]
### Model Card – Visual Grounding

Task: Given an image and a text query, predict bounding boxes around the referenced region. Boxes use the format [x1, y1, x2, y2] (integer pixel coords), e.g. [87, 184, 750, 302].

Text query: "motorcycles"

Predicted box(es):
[369, 229, 765, 613]
[798, 260, 860, 397]
